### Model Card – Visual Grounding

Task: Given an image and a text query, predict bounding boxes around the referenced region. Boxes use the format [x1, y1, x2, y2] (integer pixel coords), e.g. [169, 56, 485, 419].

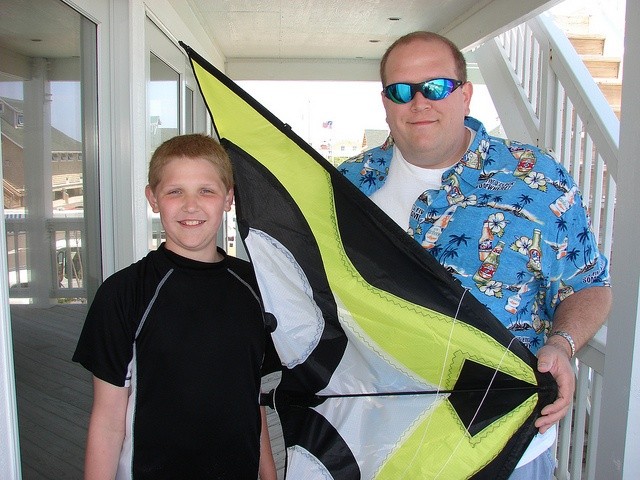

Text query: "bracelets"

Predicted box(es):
[546, 330, 576, 361]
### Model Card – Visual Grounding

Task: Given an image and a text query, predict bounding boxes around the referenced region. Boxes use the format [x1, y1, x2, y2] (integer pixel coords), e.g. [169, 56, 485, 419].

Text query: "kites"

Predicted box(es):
[177, 38, 559, 479]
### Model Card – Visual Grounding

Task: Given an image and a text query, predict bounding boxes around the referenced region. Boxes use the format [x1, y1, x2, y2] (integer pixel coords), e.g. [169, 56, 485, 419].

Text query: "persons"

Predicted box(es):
[332, 30, 610, 479]
[72, 135, 277, 480]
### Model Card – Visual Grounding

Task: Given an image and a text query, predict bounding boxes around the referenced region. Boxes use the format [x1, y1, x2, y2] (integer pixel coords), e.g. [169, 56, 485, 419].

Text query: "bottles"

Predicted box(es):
[504, 282, 528, 315]
[512, 149, 538, 177]
[532, 319, 546, 334]
[556, 235, 569, 260]
[460, 149, 484, 170]
[478, 220, 494, 261]
[446, 186, 464, 206]
[421, 204, 458, 250]
[558, 280, 574, 301]
[506, 143, 525, 160]
[549, 186, 583, 218]
[528, 228, 543, 272]
[472, 240, 506, 286]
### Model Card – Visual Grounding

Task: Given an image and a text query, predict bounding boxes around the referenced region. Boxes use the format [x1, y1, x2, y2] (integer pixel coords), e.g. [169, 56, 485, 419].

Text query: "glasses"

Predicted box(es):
[383, 79, 462, 104]
